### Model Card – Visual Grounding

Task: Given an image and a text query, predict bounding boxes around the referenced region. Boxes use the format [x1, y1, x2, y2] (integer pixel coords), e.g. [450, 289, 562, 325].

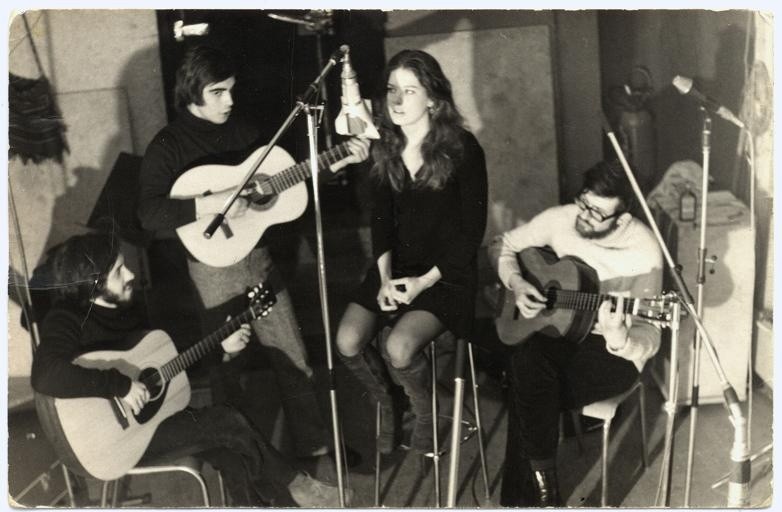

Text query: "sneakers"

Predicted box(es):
[288, 468, 353, 509]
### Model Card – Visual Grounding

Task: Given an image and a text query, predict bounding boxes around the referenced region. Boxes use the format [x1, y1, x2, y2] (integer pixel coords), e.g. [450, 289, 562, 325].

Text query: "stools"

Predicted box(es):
[98, 386, 229, 507]
[370, 303, 496, 506]
[566, 373, 650, 508]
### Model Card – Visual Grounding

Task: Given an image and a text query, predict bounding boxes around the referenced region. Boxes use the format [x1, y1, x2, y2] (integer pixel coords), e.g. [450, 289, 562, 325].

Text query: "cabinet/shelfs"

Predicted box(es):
[648, 189, 756, 404]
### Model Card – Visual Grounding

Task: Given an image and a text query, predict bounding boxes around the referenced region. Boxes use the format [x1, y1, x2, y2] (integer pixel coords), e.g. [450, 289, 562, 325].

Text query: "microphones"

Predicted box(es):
[727, 417, 751, 508]
[334, 64, 381, 140]
[672, 74, 743, 128]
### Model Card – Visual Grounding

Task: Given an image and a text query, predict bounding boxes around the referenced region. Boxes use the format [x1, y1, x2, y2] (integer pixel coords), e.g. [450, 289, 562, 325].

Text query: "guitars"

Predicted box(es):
[496, 248, 676, 348]
[32, 284, 279, 485]
[171, 140, 363, 267]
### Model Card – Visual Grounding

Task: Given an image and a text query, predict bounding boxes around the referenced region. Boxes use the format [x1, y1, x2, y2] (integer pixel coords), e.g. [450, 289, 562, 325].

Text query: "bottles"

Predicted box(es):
[678, 179, 697, 224]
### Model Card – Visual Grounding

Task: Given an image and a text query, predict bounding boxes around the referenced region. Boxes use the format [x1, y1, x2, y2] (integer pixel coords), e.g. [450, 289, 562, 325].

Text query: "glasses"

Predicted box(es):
[575, 195, 615, 222]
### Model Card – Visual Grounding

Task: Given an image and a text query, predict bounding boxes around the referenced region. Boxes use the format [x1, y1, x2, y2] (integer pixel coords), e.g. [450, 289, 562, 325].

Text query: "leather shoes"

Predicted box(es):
[531, 467, 563, 507]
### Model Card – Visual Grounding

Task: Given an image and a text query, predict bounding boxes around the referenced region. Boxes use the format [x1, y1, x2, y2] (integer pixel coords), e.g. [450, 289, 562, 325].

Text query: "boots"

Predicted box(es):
[384, 350, 439, 454]
[333, 344, 409, 455]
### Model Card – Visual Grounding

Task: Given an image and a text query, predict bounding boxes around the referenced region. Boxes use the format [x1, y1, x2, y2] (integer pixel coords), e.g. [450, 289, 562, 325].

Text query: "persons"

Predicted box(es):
[488, 159, 665, 507]
[334, 48, 488, 463]
[29, 231, 354, 507]
[135, 42, 333, 459]
